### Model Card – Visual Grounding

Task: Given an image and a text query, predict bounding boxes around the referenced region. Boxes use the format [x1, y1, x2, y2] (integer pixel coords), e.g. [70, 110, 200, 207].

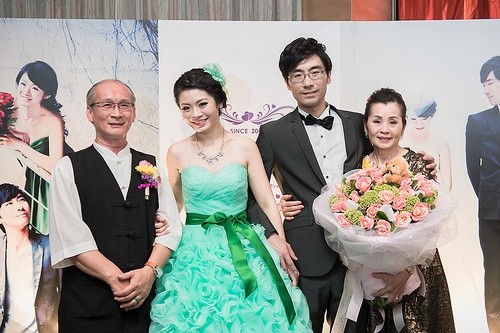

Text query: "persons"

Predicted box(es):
[148, 64, 313, 333]
[0, 61, 74, 236]
[280, 88, 455, 333]
[465, 55, 500, 333]
[49, 79, 182, 333]
[247, 37, 376, 333]
[0, 183, 61, 333]
[408, 88, 482, 333]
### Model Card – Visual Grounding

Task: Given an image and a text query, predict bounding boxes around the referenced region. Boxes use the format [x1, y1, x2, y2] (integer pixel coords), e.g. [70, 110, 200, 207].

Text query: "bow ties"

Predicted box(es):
[297, 112, 335, 131]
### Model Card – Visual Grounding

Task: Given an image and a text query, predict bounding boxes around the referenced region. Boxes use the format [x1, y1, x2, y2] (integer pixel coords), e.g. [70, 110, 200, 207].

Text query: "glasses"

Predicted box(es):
[287, 68, 326, 82]
[89, 100, 134, 111]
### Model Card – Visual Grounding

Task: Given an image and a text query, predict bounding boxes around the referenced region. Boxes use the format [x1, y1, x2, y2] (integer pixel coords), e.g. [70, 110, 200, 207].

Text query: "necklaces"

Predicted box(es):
[195, 127, 224, 163]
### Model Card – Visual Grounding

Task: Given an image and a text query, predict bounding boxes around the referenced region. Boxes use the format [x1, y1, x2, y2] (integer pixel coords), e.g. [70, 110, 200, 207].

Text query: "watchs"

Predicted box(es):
[146, 261, 163, 279]
[406, 266, 415, 275]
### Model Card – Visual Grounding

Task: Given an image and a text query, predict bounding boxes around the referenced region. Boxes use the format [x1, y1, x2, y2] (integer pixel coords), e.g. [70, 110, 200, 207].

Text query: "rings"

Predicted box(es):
[395, 296, 399, 301]
[134, 296, 140, 303]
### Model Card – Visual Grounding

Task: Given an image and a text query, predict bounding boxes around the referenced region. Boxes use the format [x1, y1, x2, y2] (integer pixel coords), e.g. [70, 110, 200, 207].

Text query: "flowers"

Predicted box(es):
[328, 169, 443, 305]
[0, 91, 25, 167]
[135, 159, 160, 199]
[201, 62, 228, 93]
